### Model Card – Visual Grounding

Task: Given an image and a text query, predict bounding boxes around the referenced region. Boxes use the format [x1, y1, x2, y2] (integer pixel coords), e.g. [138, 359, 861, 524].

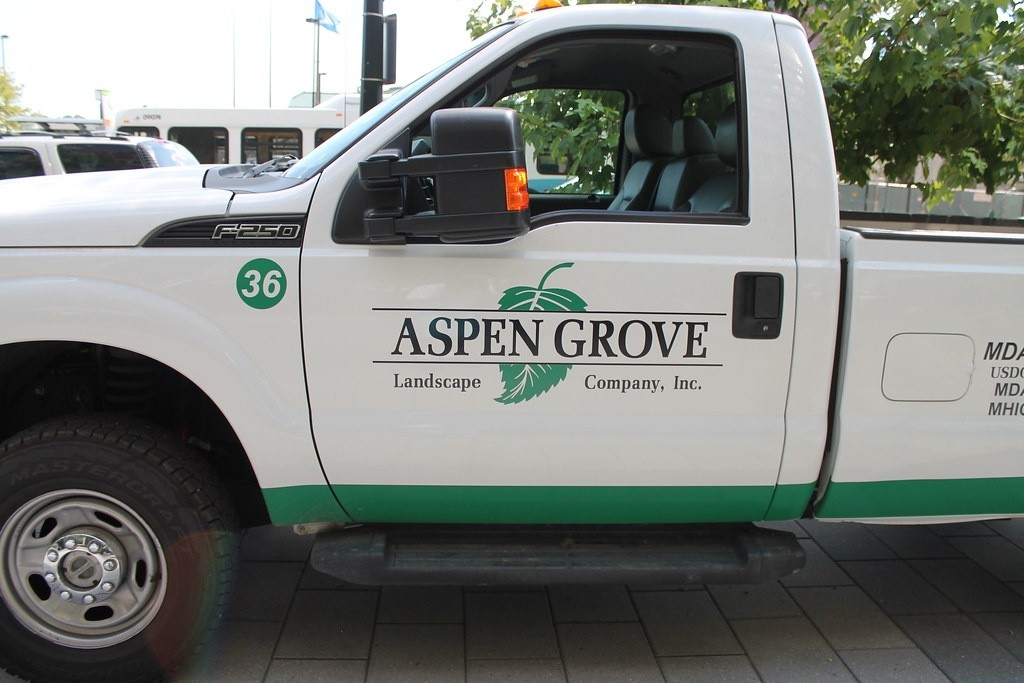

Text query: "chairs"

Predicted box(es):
[645, 115, 724, 212]
[671, 100, 740, 214]
[605, 105, 672, 212]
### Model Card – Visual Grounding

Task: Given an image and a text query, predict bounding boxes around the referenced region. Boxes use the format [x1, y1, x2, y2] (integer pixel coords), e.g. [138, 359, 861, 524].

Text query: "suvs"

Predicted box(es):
[0, 114, 201, 185]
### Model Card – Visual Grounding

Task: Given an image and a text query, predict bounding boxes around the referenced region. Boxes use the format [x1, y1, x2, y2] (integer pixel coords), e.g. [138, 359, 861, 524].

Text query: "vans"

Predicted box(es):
[103, 104, 348, 178]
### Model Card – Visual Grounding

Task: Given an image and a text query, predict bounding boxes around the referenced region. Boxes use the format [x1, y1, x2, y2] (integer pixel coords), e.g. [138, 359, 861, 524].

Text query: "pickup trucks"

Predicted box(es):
[0, 6, 1024, 683]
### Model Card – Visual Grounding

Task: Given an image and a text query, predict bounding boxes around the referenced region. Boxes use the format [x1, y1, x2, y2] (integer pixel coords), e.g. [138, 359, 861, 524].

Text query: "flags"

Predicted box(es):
[316, 0, 340, 33]
